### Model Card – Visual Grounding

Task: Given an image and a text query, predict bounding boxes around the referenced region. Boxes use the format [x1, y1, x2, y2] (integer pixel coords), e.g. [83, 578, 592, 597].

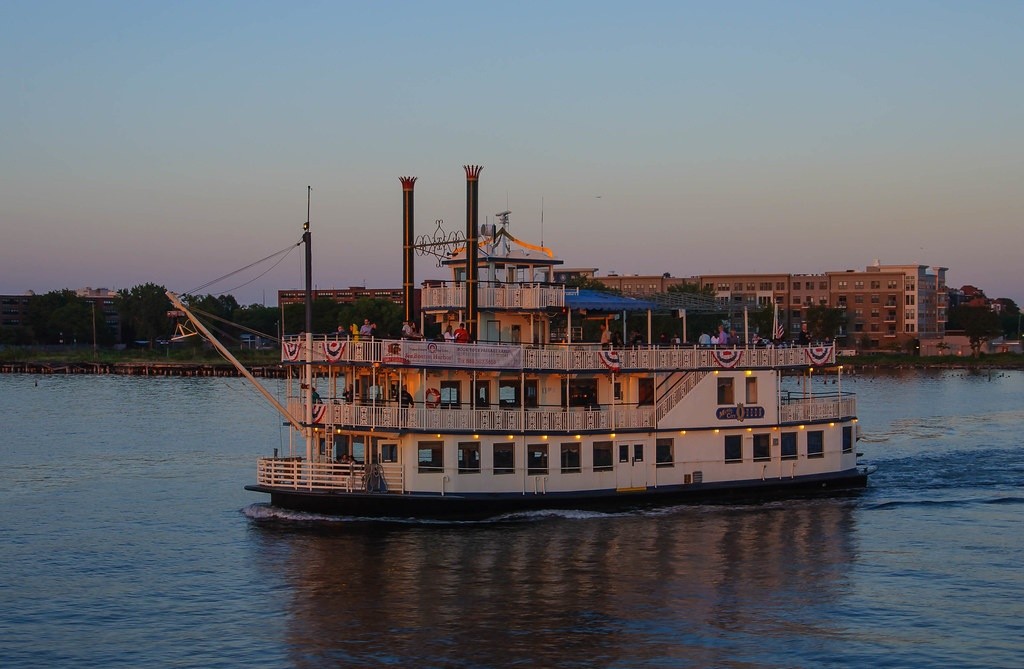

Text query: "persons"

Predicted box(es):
[699, 325, 738, 347]
[454, 323, 470, 343]
[370, 324, 378, 338]
[480, 397, 485, 407]
[350, 324, 359, 341]
[601, 324, 624, 348]
[752, 333, 786, 349]
[327, 454, 357, 476]
[799, 321, 812, 345]
[360, 319, 372, 338]
[396, 386, 414, 427]
[630, 330, 643, 347]
[401, 321, 420, 340]
[343, 384, 353, 402]
[312, 387, 323, 404]
[444, 325, 454, 342]
[495, 275, 500, 288]
[334, 326, 351, 336]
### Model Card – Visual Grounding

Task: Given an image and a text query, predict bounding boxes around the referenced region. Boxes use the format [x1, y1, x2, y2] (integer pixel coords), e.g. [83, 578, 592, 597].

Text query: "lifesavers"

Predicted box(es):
[424, 387, 442, 409]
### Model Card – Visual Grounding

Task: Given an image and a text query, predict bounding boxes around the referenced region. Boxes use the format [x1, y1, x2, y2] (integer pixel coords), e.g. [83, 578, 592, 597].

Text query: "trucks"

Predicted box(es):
[835, 350, 856, 357]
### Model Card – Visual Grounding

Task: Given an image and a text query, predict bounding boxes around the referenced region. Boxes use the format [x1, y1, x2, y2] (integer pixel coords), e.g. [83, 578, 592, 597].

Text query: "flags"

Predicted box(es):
[778, 323, 784, 336]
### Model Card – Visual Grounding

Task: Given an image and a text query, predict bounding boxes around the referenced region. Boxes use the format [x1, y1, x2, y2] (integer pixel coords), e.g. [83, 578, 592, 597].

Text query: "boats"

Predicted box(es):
[164, 166, 880, 522]
